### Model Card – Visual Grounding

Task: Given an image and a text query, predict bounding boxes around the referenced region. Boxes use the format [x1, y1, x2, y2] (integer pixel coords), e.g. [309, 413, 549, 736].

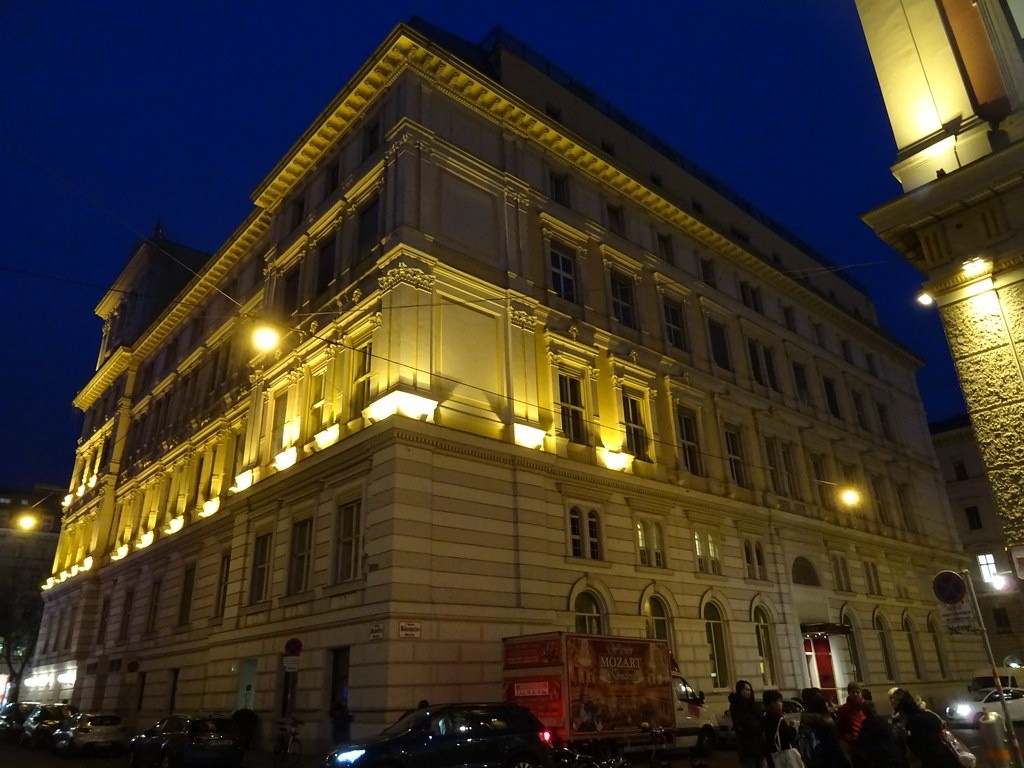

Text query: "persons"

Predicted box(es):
[727, 679, 975, 767]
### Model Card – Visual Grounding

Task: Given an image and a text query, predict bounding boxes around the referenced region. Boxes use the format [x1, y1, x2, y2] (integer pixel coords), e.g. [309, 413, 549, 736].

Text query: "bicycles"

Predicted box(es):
[273, 717, 305, 766]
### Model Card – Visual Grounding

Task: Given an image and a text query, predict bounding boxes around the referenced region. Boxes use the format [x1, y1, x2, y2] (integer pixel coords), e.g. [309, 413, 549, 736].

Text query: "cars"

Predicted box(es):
[723, 700, 803, 743]
[947, 687, 1024, 728]
[319, 701, 601, 768]
[126, 709, 242, 768]
[45, 712, 126, 757]
[0, 702, 75, 751]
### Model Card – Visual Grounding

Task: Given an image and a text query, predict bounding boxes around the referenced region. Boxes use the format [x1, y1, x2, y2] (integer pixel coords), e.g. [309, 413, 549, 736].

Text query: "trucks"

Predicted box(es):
[501, 630, 719, 759]
[967, 666, 1024, 693]
